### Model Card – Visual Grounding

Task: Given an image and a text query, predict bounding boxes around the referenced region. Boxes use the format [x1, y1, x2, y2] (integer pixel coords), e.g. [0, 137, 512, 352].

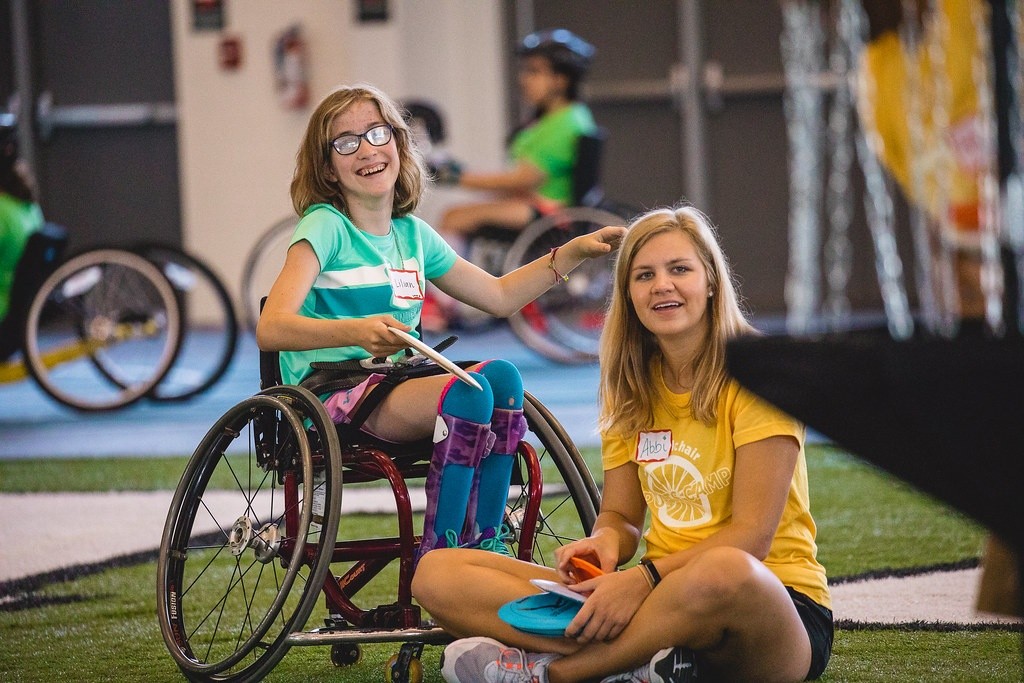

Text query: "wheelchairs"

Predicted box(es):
[447, 194, 644, 368]
[0, 220, 243, 411]
[153, 294, 608, 683]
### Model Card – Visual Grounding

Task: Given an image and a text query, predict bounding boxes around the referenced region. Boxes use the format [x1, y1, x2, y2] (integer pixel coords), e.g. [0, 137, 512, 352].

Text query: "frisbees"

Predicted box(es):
[386, 326, 485, 392]
[497, 557, 607, 637]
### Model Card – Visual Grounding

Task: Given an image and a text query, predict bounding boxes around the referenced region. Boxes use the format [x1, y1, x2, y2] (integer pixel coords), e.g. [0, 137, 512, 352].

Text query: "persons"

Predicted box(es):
[409, 207, 835, 683]
[256, 86, 629, 591]
[0, 127, 45, 322]
[442, 28, 598, 236]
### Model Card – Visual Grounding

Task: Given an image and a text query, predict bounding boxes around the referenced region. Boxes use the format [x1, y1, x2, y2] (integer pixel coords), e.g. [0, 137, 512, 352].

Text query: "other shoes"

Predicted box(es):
[422, 295, 448, 334]
[410, 529, 466, 627]
[474, 523, 511, 556]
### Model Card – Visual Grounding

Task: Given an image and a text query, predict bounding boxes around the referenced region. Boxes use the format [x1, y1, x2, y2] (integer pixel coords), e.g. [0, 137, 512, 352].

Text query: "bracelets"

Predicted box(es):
[549, 247, 568, 282]
[637, 558, 662, 591]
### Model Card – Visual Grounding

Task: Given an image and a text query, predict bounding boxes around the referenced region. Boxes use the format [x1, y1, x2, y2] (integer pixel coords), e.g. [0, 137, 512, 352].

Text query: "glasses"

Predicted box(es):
[327, 125, 397, 155]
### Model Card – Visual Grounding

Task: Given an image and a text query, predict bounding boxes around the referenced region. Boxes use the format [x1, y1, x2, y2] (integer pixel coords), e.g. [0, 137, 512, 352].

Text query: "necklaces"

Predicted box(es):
[346, 209, 404, 270]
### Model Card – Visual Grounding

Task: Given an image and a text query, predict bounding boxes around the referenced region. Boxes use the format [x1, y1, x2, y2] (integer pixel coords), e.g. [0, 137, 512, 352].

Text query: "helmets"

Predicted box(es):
[517, 29, 595, 73]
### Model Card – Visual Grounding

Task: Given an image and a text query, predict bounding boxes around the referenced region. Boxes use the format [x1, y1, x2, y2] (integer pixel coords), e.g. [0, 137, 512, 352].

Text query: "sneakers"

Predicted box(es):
[599, 648, 697, 683]
[439, 636, 562, 683]
